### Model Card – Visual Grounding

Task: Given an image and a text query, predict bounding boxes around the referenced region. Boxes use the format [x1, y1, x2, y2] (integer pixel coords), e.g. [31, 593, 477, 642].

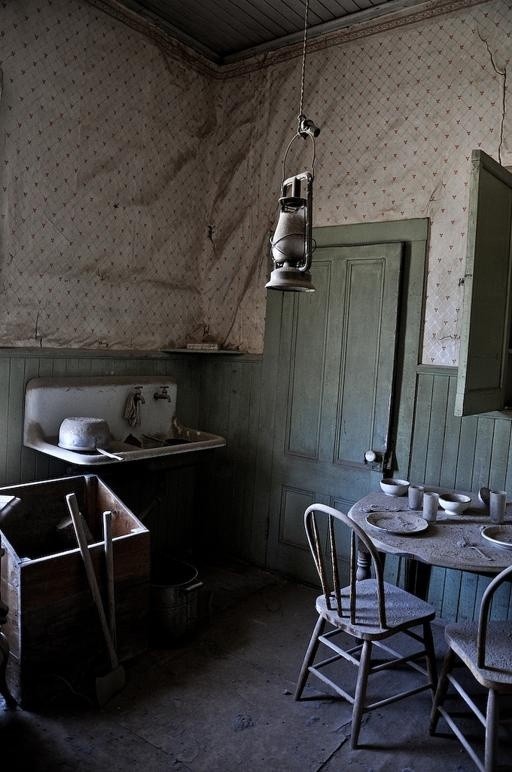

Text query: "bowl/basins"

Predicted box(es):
[380, 478, 410, 498]
[439, 493, 472, 516]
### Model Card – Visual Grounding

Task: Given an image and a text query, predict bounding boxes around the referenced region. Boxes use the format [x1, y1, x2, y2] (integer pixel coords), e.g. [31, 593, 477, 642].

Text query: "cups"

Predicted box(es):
[423, 492, 441, 521]
[408, 485, 424, 511]
[489, 491, 508, 525]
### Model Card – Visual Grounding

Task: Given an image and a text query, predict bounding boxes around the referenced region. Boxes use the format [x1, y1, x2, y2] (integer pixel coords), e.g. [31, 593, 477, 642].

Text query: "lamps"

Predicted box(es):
[262, 0, 321, 293]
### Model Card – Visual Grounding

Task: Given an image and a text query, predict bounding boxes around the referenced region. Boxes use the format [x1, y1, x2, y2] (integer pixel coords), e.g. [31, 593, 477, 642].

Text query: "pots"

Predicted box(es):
[58, 416, 128, 461]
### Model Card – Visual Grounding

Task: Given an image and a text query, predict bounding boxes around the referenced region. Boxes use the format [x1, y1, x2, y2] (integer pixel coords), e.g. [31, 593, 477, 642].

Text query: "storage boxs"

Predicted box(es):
[0, 471, 154, 714]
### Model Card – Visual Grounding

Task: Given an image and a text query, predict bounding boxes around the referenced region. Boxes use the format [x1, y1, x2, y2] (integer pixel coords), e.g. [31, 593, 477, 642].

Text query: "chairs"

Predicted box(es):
[427, 563, 512, 772]
[294, 503, 439, 750]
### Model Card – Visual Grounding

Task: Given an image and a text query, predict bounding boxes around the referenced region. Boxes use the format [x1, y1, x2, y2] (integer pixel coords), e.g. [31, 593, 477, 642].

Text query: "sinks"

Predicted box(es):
[115, 376, 226, 456]
[23, 375, 140, 466]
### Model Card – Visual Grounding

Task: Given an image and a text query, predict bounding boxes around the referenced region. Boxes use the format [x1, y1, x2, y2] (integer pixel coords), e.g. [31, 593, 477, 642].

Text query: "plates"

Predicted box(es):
[482, 524, 512, 552]
[366, 512, 430, 535]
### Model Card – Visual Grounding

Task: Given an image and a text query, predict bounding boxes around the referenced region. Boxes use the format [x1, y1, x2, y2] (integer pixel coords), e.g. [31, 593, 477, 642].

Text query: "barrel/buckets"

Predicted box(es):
[148, 558, 204, 643]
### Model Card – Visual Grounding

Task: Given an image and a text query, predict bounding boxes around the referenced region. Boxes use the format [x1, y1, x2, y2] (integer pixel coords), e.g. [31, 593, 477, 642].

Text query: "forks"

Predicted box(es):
[460, 535, 494, 563]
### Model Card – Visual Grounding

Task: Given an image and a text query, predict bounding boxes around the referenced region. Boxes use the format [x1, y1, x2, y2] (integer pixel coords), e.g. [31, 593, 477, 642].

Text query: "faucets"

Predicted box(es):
[135, 385, 146, 404]
[154, 386, 171, 403]
